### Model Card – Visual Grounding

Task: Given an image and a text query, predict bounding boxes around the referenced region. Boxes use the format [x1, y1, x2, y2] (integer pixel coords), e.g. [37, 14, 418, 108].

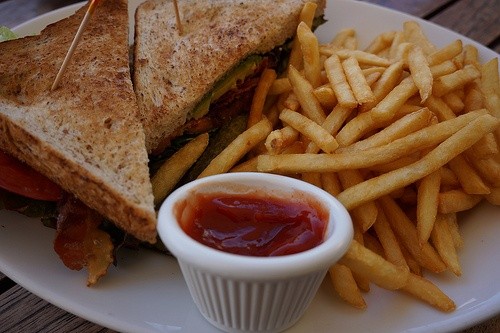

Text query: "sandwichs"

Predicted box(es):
[1, 0, 326, 288]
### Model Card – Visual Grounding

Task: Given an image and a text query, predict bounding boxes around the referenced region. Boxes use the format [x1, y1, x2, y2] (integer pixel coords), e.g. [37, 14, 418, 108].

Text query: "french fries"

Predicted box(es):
[153, 1, 500, 312]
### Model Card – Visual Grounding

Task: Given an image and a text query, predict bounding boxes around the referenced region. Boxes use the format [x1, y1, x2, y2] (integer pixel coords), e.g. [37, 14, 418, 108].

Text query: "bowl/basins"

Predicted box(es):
[156, 171, 355, 333]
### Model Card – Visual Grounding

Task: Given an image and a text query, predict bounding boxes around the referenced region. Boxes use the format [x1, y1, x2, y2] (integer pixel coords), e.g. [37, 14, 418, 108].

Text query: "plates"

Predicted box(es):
[1, 1, 500, 333]
